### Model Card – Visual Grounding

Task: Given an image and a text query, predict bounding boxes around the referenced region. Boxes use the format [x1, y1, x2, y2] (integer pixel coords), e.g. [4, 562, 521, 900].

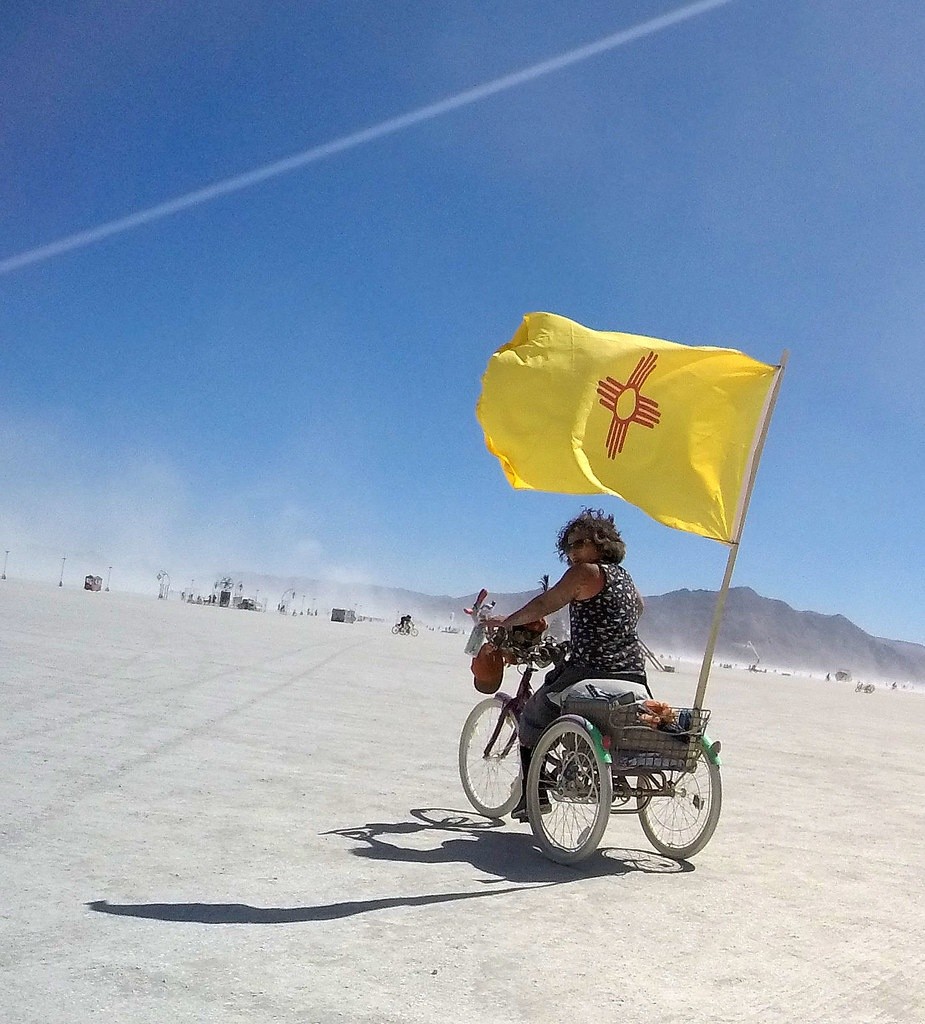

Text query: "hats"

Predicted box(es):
[471, 641, 503, 694]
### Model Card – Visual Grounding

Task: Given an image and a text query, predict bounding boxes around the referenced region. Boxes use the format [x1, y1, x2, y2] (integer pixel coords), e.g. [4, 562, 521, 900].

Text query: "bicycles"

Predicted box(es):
[391, 623, 419, 637]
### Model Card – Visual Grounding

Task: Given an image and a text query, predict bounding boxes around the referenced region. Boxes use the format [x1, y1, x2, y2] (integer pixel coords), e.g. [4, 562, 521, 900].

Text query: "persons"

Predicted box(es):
[180, 592, 217, 605]
[478, 507, 647, 818]
[395, 615, 413, 634]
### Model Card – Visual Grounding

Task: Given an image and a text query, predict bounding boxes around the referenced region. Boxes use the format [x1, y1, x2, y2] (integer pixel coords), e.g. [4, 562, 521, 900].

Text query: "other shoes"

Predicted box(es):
[510, 777, 552, 819]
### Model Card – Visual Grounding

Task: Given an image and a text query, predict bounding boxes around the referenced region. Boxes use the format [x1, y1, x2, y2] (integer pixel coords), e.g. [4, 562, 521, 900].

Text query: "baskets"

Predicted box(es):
[563, 696, 711, 774]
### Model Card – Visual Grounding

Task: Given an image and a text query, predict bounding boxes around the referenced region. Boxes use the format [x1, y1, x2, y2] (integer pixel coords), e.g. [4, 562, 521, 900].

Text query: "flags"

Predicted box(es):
[478, 310, 781, 544]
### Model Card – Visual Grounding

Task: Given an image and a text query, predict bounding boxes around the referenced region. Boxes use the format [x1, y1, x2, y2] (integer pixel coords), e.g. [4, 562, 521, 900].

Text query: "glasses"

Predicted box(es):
[562, 538, 595, 555]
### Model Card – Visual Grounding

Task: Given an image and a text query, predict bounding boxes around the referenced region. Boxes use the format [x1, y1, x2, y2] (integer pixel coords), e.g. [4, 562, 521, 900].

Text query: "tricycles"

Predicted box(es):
[458, 587, 722, 865]
